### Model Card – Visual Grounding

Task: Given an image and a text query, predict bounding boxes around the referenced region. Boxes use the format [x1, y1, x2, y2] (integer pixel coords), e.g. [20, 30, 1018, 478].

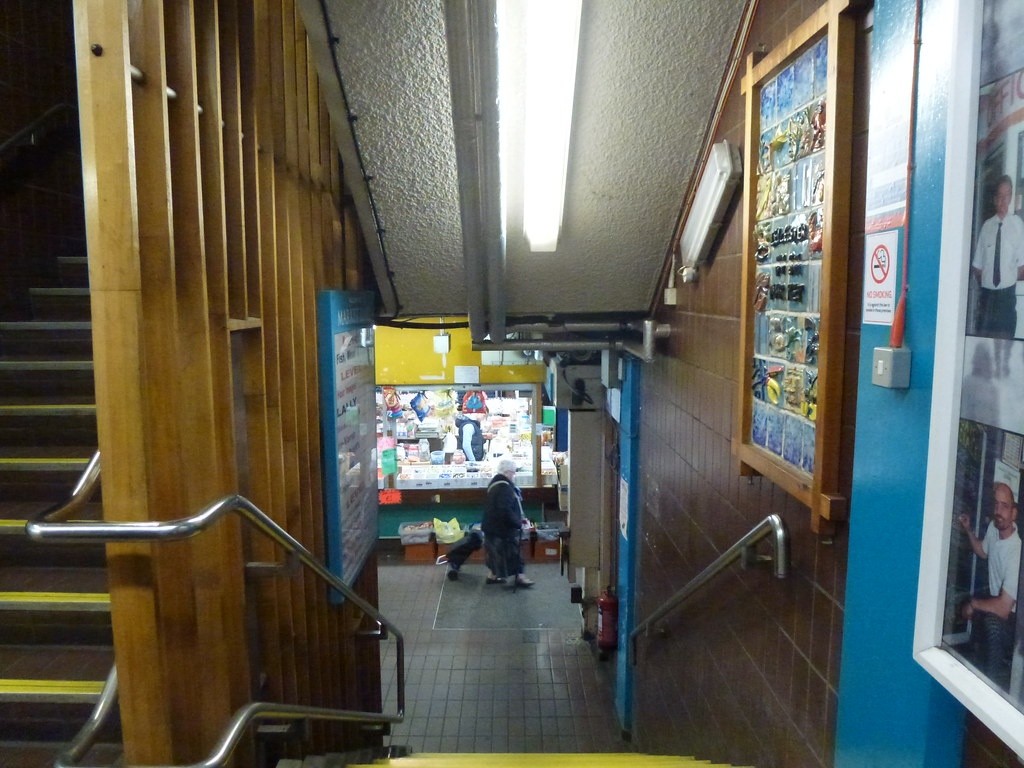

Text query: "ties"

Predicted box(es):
[993, 223, 1002, 287]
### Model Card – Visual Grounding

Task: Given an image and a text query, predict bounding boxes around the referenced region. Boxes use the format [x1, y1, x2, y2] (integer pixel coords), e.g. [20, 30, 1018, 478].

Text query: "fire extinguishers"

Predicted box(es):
[597, 584, 618, 648]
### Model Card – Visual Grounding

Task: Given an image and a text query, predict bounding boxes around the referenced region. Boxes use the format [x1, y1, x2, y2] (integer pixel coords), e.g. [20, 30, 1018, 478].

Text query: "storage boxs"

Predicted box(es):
[398, 520, 559, 562]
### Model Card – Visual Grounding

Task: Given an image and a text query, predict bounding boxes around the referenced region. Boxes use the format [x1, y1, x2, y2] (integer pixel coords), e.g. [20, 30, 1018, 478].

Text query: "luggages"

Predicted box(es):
[436, 530, 483, 580]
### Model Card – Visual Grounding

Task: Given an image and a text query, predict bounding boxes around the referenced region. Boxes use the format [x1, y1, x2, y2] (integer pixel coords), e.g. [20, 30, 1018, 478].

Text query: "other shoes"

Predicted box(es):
[514, 579, 534, 587]
[485, 576, 507, 584]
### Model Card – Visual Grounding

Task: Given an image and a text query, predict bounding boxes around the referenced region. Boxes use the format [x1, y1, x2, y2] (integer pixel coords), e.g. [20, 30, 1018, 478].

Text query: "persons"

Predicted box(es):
[960, 482, 1021, 695]
[480, 459, 535, 587]
[973, 174, 1024, 338]
[456, 406, 496, 462]
[961, 337, 1013, 426]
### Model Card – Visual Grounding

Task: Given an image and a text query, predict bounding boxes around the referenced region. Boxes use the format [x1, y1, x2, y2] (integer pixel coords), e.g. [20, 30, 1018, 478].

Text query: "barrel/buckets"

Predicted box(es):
[431, 450, 445, 465]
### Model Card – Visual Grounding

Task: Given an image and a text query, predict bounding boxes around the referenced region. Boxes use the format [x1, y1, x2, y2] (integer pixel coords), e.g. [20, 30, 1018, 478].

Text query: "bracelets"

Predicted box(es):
[970, 601, 973, 609]
